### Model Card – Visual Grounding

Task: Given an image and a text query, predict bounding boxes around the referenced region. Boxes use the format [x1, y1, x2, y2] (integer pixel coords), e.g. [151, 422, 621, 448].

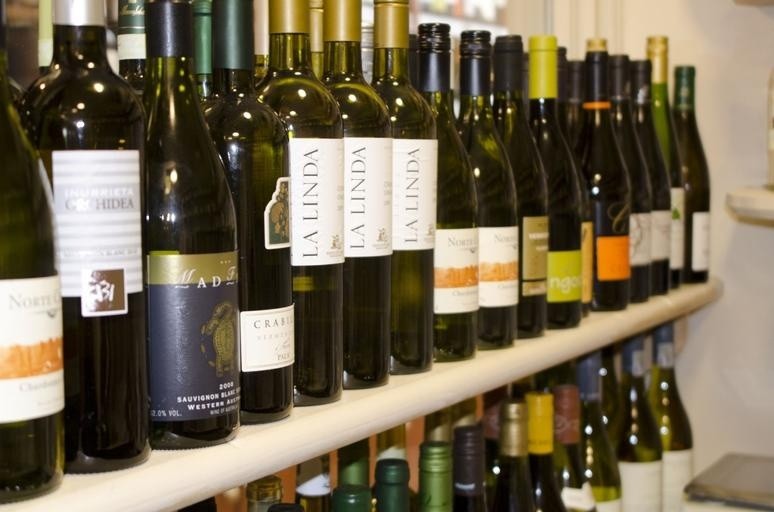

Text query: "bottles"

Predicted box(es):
[0, 0, 711, 512]
